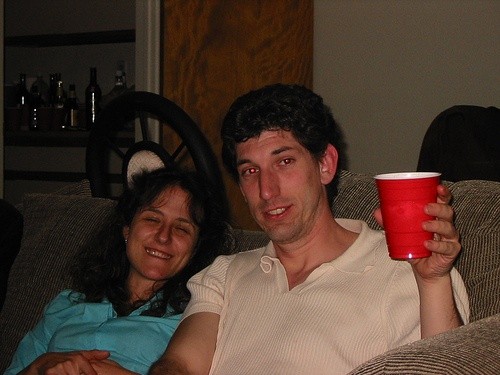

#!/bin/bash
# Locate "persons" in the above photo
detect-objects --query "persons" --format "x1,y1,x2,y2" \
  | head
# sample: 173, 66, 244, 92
3, 166, 231, 375
148, 85, 470, 375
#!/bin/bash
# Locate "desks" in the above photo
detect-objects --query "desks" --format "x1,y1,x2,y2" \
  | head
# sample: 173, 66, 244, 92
3, 129, 134, 194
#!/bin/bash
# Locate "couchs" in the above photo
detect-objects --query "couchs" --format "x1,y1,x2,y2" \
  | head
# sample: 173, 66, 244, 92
11, 171, 500, 375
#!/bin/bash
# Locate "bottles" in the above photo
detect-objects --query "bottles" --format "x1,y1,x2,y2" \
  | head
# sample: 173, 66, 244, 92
16, 73, 28, 107
85, 66, 102, 128
67, 84, 79, 129
107, 69, 131, 100
30, 72, 50, 131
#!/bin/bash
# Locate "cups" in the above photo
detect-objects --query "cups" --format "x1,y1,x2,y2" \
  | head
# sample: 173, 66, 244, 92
373, 171, 442, 259
